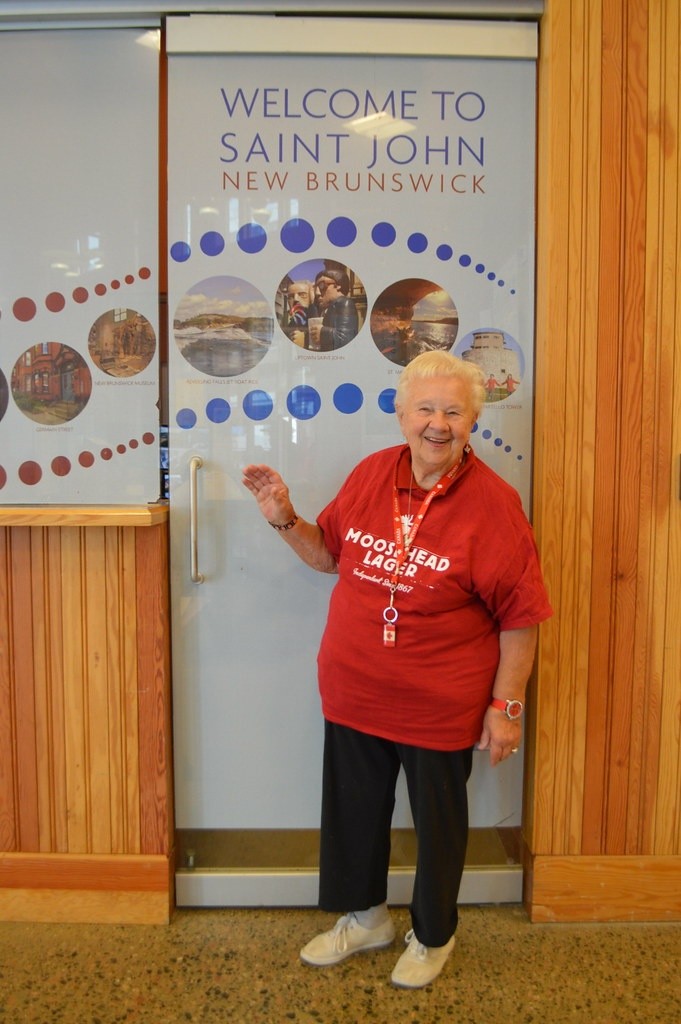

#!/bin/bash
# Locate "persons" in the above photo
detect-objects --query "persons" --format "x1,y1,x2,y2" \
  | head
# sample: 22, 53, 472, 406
484, 372, 499, 399
500, 372, 520, 396
239, 347, 555, 987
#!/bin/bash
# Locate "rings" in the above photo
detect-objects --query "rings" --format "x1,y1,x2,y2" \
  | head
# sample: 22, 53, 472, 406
512, 748, 519, 753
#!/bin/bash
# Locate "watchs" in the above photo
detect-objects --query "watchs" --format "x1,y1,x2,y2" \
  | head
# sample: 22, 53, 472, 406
491, 697, 527, 720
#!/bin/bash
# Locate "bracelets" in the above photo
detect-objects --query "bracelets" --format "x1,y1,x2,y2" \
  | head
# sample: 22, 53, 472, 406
268, 512, 298, 531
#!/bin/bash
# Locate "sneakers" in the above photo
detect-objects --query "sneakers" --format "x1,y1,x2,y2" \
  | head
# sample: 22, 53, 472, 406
391, 928, 457, 987
300, 913, 394, 965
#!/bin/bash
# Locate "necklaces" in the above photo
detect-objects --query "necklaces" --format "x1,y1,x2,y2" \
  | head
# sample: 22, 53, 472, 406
405, 456, 467, 543
